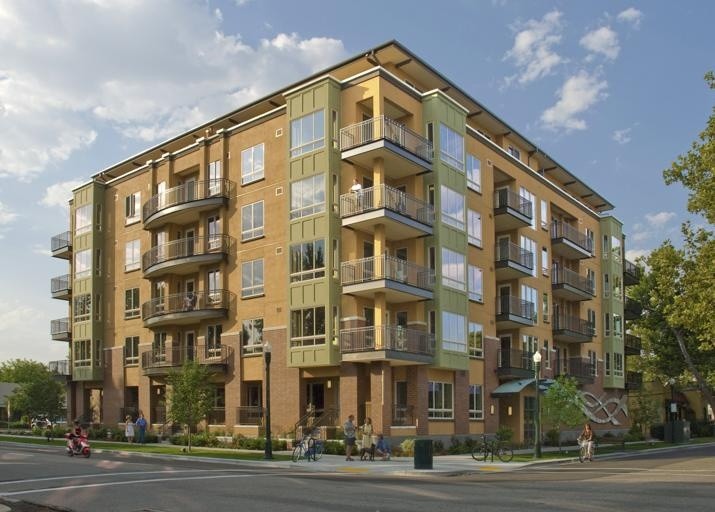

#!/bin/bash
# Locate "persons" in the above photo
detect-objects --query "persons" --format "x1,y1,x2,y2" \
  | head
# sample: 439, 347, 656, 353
124, 414, 135, 443
376, 433, 392, 461
576, 422, 594, 463
360, 417, 373, 461
44, 417, 52, 442
73, 419, 82, 451
349, 178, 362, 211
135, 411, 147, 442
342, 414, 357, 461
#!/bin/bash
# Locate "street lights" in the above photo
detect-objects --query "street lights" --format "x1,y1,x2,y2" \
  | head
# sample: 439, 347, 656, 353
667, 377, 679, 441
533, 349, 542, 458
263, 341, 274, 459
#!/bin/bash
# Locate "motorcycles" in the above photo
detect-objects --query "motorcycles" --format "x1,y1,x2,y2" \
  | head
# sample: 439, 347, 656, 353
63, 431, 93, 458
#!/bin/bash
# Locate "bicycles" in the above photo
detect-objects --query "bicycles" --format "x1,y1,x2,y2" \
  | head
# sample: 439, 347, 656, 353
471, 431, 515, 461
292, 432, 322, 462
578, 438, 596, 463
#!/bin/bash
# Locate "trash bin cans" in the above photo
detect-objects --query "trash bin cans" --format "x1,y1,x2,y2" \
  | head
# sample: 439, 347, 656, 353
414, 440, 433, 469
663, 421, 690, 443
